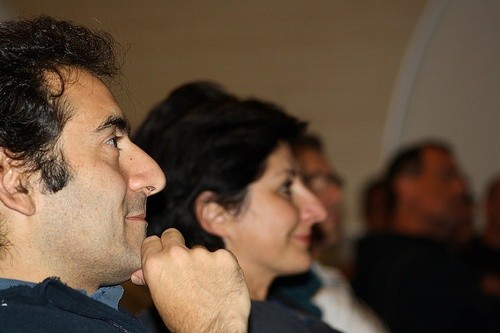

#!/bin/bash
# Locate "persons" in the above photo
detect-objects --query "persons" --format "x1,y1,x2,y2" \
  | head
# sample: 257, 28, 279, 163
263, 131, 421, 333
0, 16, 252, 333
131, 80, 344, 333
349, 138, 500, 333
